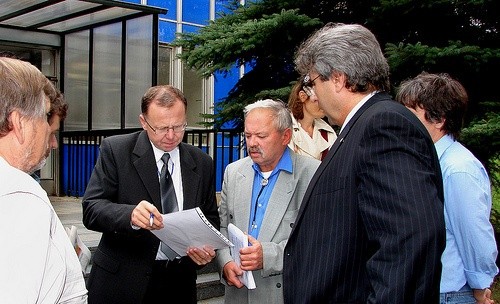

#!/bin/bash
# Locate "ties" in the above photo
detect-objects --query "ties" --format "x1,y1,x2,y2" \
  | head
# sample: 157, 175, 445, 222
159, 153, 180, 261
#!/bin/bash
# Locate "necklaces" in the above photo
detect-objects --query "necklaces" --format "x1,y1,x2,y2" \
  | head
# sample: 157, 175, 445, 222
300, 124, 313, 138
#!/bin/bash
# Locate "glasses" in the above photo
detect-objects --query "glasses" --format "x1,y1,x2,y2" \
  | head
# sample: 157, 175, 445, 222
144, 118, 187, 135
303, 74, 322, 96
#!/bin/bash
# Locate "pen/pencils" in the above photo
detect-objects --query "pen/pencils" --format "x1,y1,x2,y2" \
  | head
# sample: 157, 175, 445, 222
150, 212, 154, 227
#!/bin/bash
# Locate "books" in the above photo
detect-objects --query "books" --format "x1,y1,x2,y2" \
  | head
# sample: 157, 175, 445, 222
227, 223, 257, 289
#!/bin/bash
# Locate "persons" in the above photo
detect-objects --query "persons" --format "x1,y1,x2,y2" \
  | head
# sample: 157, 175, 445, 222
396, 71, 499, 304
81, 85, 221, 304
0, 57, 88, 304
287, 74, 338, 161
216, 99, 322, 304
283, 23, 446, 304
